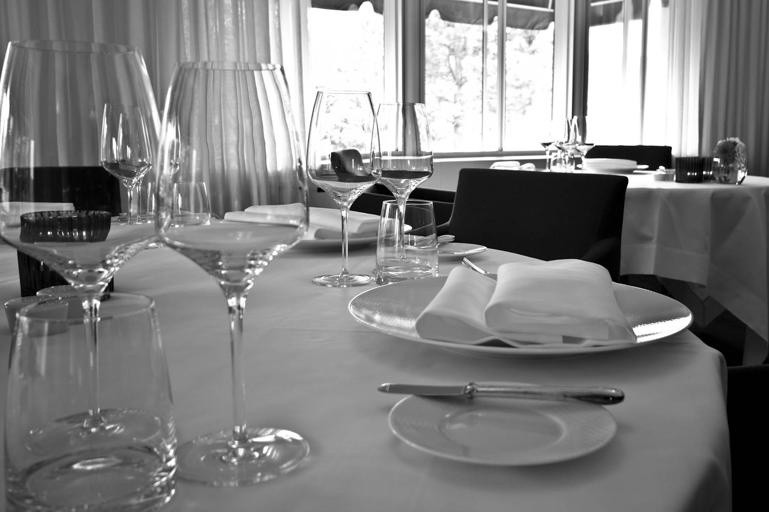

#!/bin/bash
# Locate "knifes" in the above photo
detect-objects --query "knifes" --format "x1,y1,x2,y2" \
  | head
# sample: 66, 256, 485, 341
376, 380, 625, 409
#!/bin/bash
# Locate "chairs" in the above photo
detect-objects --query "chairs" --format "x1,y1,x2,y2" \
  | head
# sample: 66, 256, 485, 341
584, 141, 673, 170
401, 166, 634, 283
0, 164, 124, 218
327, 148, 455, 228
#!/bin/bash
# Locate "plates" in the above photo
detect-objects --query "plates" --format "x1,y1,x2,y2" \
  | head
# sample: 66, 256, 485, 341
576, 163, 649, 174
436, 241, 490, 260
291, 214, 413, 250
346, 275, 695, 358
388, 394, 620, 469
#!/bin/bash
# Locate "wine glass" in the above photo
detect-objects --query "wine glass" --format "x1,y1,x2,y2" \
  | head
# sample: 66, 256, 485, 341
151, 58, 311, 485
537, 115, 596, 172
1, 34, 161, 431
304, 91, 376, 289
0, 34, 312, 510
371, 105, 444, 288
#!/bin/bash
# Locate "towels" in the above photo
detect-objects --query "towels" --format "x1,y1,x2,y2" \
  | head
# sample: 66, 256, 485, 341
224, 202, 396, 242
410, 253, 637, 349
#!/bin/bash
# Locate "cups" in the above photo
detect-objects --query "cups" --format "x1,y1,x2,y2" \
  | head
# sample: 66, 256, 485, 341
3, 293, 178, 511
376, 198, 440, 282
